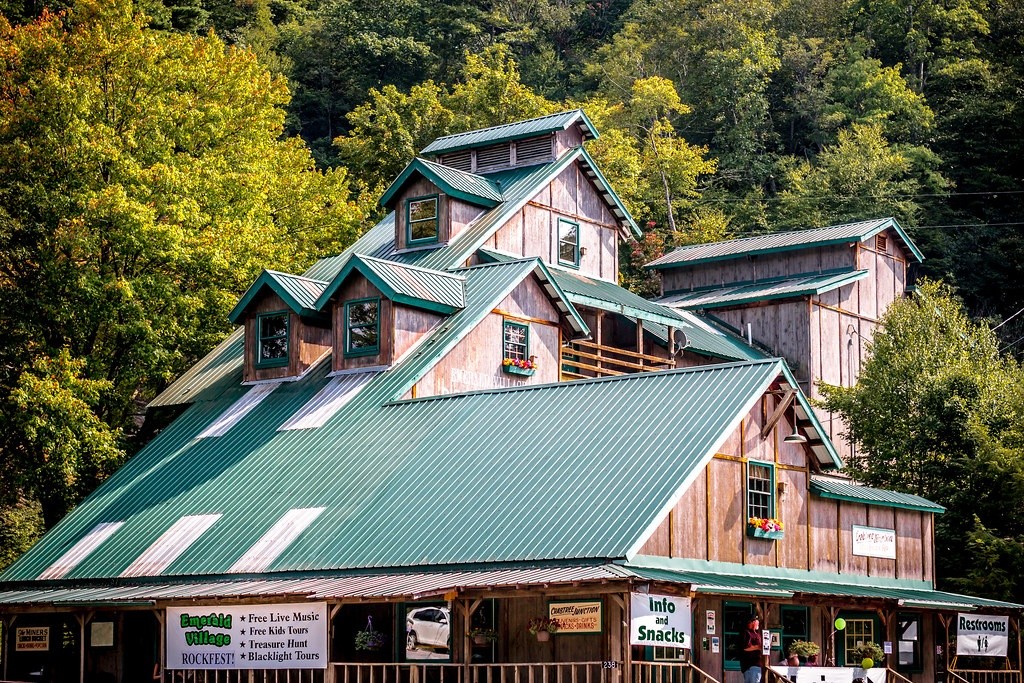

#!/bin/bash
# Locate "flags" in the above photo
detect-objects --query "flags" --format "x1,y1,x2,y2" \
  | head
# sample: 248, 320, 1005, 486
631, 592, 693, 648
957, 613, 1010, 658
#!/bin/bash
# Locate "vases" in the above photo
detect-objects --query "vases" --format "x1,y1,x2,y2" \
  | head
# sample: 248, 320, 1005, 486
473, 634, 488, 645
536, 631, 550, 641
366, 644, 380, 650
747, 526, 784, 540
504, 365, 535, 376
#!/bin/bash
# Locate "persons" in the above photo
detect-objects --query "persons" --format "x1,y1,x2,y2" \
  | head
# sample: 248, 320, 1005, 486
738, 615, 763, 683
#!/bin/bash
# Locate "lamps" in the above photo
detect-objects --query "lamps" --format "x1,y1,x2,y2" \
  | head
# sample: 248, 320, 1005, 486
783, 394, 807, 443
835, 618, 846, 630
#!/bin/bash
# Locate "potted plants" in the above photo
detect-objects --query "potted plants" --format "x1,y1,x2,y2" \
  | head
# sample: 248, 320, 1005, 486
807, 642, 820, 666
789, 639, 806, 666
853, 641, 886, 669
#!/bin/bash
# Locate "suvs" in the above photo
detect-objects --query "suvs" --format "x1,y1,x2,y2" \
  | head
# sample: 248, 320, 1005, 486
406, 607, 451, 651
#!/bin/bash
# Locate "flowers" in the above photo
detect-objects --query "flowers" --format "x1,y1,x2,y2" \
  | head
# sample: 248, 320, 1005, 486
467, 628, 497, 641
503, 359, 536, 369
527, 618, 561, 634
751, 517, 784, 533
356, 631, 384, 646
